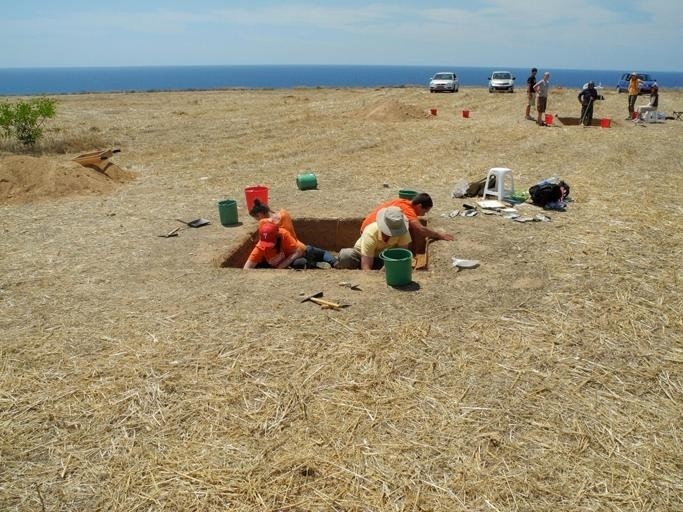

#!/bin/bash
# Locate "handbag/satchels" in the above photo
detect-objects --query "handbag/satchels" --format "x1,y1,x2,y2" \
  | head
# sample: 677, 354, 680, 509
529, 182, 562, 204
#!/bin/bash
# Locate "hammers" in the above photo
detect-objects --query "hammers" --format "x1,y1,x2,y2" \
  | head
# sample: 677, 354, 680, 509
157, 227, 180, 238
301, 290, 340, 308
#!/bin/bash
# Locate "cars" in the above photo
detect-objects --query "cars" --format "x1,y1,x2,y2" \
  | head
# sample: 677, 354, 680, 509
616, 72, 658, 95
488, 71, 517, 93
430, 71, 459, 94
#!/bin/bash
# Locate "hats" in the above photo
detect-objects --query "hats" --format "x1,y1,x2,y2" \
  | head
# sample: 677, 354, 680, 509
376, 206, 409, 237
258, 223, 278, 248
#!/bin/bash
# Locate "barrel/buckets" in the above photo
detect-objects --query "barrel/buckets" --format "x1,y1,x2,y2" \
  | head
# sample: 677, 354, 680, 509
296, 173, 318, 190
463, 111, 469, 118
545, 114, 553, 124
246, 186, 269, 212
601, 118, 611, 128
378, 248, 413, 288
631, 112, 639, 120
431, 109, 436, 116
219, 199, 238, 225
399, 189, 417, 197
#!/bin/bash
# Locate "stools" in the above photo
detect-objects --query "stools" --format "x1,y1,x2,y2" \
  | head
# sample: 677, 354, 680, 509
645, 110, 657, 123
484, 168, 515, 200
673, 111, 683, 121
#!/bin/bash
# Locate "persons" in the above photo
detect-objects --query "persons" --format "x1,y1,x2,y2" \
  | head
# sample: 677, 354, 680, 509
335, 204, 414, 271
242, 221, 309, 269
623, 72, 646, 119
360, 191, 456, 257
523, 68, 539, 120
532, 72, 551, 126
576, 80, 598, 126
249, 197, 341, 269
634, 83, 659, 124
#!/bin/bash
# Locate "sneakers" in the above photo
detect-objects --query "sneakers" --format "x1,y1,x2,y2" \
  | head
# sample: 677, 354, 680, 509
315, 260, 331, 269
333, 261, 345, 269
526, 115, 546, 126
625, 115, 641, 123
